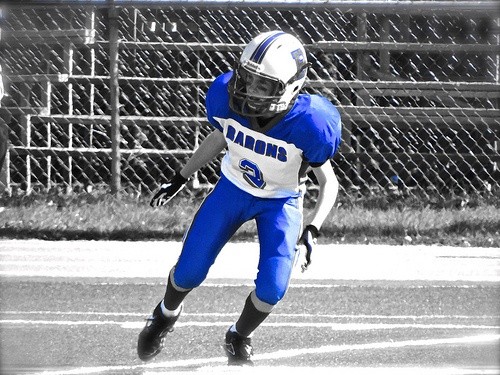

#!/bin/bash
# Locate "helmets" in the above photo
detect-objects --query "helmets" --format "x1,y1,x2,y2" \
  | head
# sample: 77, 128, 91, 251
239, 29, 308, 112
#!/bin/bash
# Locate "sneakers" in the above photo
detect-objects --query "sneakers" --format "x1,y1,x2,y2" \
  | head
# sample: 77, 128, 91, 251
137, 303, 182, 361
222, 326, 255, 367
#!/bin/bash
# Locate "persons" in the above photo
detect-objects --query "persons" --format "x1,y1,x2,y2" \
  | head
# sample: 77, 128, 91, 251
137, 30, 341, 367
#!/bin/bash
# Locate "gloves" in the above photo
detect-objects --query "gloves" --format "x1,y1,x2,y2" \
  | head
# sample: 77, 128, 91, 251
295, 225, 320, 274
151, 170, 188, 207
